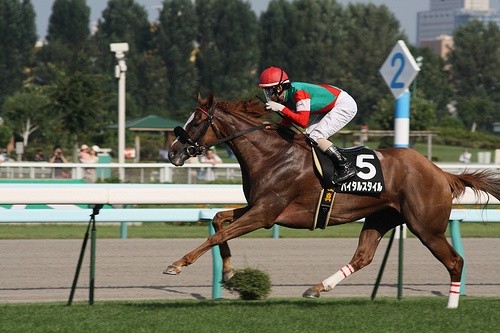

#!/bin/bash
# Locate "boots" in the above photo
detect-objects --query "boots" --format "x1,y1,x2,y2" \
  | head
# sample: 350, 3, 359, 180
323, 143, 358, 185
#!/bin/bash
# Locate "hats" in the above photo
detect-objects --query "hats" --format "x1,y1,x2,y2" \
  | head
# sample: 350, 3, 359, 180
80, 144, 89, 150
91, 145, 100, 153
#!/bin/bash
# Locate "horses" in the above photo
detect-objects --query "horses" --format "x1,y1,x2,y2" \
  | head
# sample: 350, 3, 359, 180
162, 92, 500, 309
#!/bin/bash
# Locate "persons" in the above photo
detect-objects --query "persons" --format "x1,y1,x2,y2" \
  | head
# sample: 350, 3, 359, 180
198, 149, 222, 181
32, 143, 100, 184
256, 67, 358, 186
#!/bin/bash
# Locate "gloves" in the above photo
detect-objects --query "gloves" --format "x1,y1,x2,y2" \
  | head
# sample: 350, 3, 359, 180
266, 101, 285, 111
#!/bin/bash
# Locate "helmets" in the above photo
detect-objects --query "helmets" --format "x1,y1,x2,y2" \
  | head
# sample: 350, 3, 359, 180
259, 66, 290, 88
